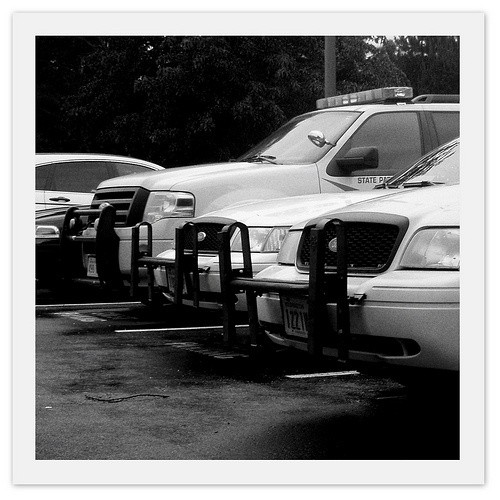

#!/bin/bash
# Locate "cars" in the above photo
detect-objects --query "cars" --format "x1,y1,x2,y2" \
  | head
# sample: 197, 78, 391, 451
150, 138, 458, 310
254, 182, 461, 371
34, 152, 166, 246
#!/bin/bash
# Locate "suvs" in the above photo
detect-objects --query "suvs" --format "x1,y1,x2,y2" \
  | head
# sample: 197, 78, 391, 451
82, 87, 459, 282
